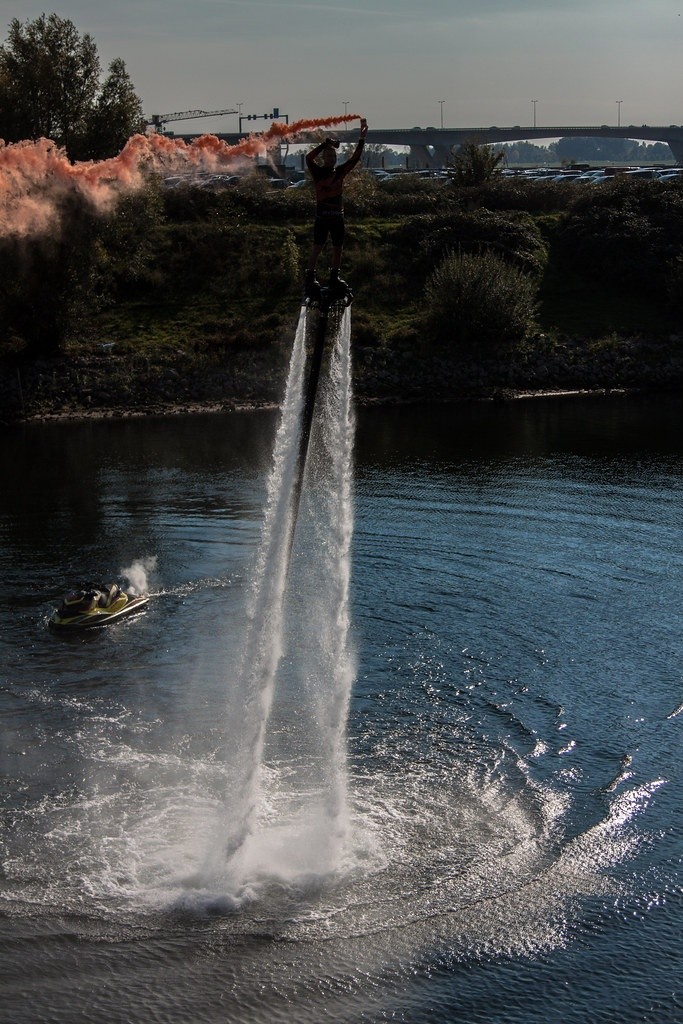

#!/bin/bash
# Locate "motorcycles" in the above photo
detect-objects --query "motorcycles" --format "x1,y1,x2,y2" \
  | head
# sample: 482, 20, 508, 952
47, 580, 151, 633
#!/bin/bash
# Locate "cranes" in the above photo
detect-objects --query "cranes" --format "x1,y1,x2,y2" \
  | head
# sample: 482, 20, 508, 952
146, 108, 239, 135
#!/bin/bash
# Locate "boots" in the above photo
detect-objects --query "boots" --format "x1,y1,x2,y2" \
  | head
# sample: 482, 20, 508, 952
328, 266, 348, 291
303, 269, 321, 290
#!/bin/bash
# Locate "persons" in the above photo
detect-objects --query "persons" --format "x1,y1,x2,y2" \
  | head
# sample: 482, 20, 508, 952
304, 124, 370, 307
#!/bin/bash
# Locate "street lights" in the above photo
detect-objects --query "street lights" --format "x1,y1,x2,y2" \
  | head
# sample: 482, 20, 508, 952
532, 100, 539, 127
342, 101, 352, 131
616, 100, 623, 127
438, 100, 447, 128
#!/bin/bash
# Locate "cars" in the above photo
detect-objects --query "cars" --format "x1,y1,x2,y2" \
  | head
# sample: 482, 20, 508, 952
162, 163, 683, 190
